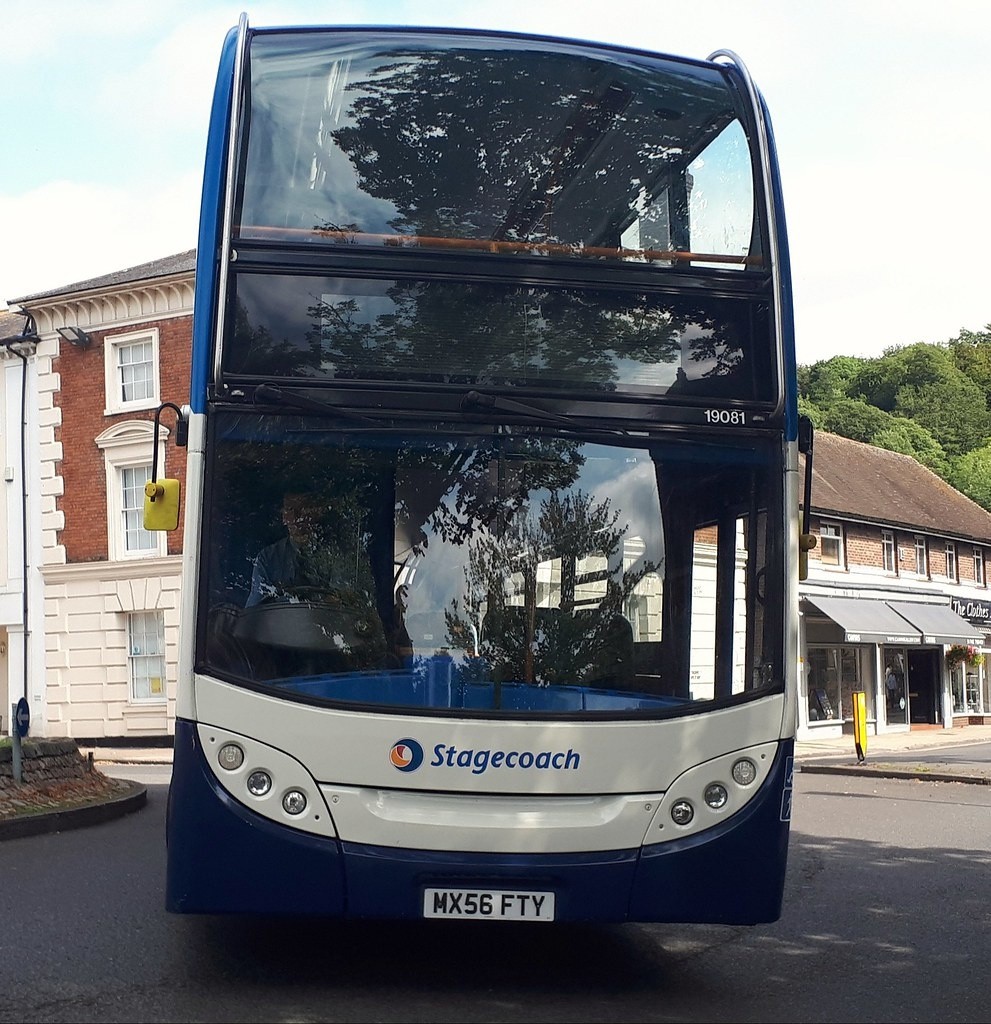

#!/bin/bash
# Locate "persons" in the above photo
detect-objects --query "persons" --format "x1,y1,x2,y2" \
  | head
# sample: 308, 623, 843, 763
245, 486, 376, 608
884, 666, 899, 709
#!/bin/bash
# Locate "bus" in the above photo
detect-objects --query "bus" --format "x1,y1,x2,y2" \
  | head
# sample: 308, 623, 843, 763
141, 11, 817, 927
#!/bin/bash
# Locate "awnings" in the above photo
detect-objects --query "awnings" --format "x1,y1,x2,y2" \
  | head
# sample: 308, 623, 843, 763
885, 600, 986, 646
804, 597, 922, 646
